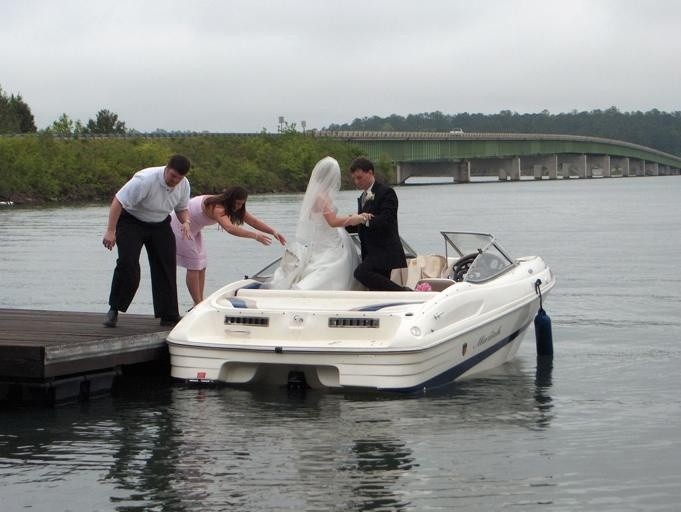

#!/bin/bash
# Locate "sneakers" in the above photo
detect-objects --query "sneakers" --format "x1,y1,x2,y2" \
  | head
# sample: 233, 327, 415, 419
159, 317, 182, 327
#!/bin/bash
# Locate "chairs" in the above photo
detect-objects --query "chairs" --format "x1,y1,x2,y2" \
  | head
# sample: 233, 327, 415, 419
414, 278, 456, 293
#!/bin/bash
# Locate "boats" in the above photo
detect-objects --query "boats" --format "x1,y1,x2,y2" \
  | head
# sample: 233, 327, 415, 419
166, 232, 556, 393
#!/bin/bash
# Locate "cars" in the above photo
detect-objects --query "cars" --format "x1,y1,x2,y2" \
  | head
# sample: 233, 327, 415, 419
450, 128, 463, 135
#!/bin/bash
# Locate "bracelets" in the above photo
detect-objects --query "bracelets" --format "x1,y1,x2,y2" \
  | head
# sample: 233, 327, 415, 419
186, 219, 194, 225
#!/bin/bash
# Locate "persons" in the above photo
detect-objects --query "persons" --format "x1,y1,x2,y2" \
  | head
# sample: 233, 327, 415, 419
101, 154, 193, 328
344, 157, 415, 291
258, 156, 375, 291
170, 185, 289, 306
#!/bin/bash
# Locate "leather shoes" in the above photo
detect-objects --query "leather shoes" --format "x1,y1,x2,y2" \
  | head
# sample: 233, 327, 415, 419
103, 306, 119, 328
